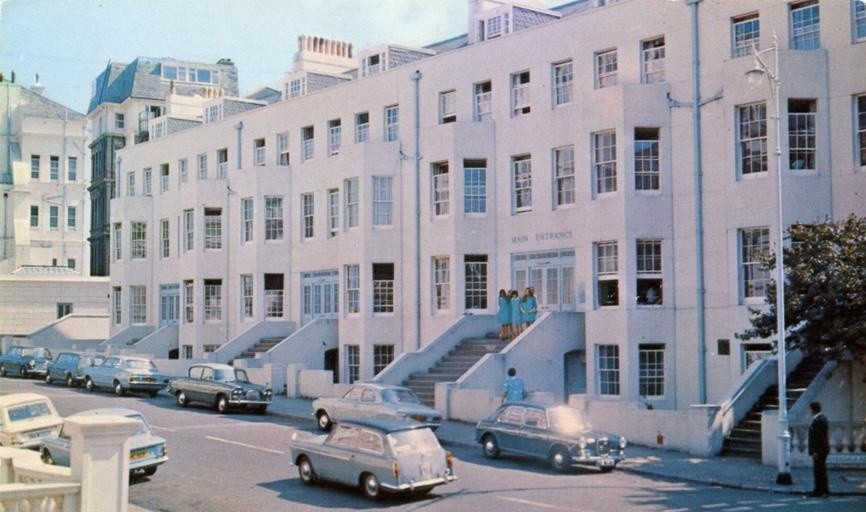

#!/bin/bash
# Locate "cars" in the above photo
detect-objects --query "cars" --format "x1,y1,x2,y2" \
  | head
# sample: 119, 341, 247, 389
0, 342, 172, 487
164, 360, 275, 411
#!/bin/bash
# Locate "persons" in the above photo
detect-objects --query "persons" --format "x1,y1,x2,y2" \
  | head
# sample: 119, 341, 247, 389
496, 286, 539, 340
499, 367, 527, 405
808, 401, 830, 497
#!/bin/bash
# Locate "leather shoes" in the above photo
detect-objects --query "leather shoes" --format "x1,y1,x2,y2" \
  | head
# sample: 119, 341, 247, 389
807, 491, 832, 498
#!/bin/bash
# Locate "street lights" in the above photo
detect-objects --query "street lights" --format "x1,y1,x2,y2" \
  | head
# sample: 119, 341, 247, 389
743, 28, 798, 489
